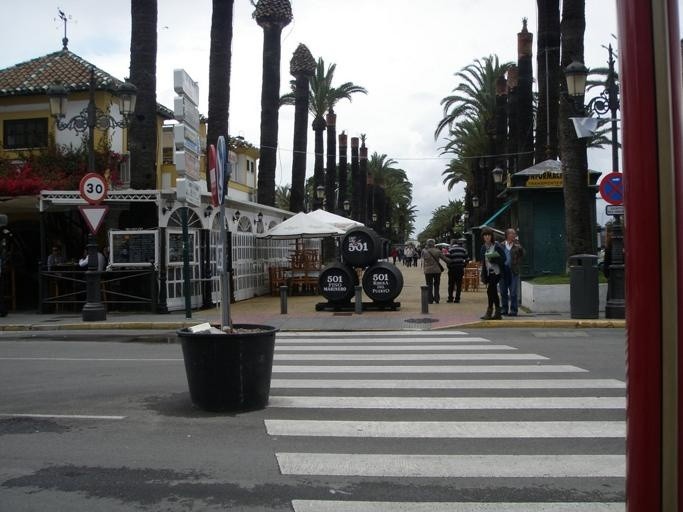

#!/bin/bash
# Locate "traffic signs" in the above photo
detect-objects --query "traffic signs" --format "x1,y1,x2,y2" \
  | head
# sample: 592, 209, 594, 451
80, 173, 108, 205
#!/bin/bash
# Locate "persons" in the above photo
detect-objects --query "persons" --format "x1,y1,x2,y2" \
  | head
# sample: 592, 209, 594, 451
390, 238, 468, 304
499, 228, 523, 316
47, 239, 109, 270
477, 228, 506, 320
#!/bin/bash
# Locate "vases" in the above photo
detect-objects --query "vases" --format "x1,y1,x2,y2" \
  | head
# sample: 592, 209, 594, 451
177, 322, 280, 413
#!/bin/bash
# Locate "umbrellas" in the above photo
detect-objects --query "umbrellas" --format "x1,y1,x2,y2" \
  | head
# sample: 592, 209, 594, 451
254, 212, 345, 277
306, 209, 363, 229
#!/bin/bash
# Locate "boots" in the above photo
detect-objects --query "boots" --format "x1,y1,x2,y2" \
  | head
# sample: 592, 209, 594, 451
480, 306, 503, 320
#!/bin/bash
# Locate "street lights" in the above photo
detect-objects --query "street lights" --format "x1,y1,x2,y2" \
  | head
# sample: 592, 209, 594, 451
562, 42, 622, 319
491, 167, 508, 191
304, 180, 391, 230
457, 196, 481, 227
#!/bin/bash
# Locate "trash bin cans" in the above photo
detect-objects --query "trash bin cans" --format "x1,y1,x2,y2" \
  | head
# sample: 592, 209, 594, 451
570, 254, 600, 318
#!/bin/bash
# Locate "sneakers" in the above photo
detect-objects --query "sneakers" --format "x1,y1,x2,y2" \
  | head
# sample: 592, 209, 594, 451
428, 296, 460, 304
501, 307, 518, 316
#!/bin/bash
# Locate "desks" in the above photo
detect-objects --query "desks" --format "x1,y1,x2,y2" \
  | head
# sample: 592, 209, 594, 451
53, 262, 80, 313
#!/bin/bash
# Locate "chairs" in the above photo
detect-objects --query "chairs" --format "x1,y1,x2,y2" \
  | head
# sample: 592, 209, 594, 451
458, 260, 487, 292
269, 261, 326, 296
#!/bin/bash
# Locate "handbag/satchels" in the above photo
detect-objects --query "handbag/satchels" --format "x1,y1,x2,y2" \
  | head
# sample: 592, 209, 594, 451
438, 262, 444, 272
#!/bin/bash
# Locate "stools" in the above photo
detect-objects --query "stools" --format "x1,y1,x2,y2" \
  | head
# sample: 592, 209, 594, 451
46, 276, 108, 315
